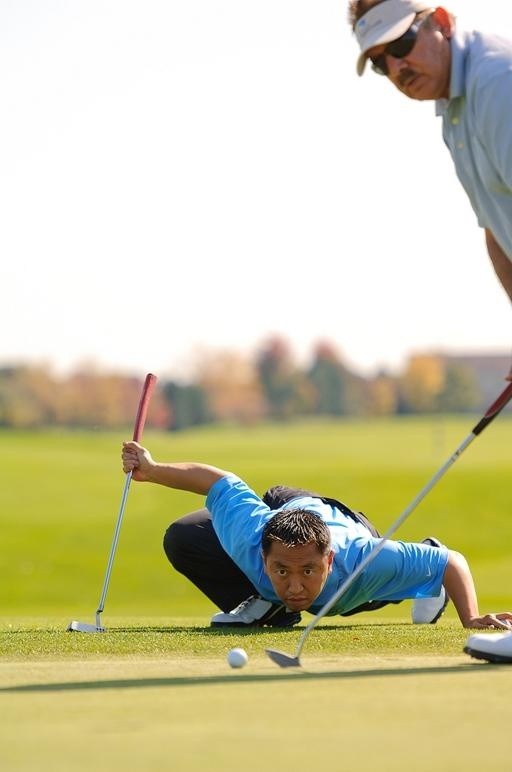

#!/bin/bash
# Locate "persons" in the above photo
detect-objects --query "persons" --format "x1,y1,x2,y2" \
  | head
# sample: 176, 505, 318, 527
121, 440, 511, 629
346, 1, 511, 663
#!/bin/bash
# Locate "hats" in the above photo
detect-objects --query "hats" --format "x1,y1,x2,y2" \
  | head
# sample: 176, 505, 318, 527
354, 0, 429, 76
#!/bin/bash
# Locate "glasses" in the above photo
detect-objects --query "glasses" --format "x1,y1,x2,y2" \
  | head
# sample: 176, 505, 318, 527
370, 16, 428, 76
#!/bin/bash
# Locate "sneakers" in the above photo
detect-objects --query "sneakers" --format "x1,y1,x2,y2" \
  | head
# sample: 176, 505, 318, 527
210, 595, 302, 628
463, 628, 512, 663
411, 537, 450, 625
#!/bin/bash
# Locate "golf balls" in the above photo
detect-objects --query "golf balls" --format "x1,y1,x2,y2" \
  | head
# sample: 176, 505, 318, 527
229, 648, 246, 669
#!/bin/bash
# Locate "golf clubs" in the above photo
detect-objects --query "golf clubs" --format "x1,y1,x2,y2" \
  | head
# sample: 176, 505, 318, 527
68, 374, 157, 633
265, 383, 512, 668
